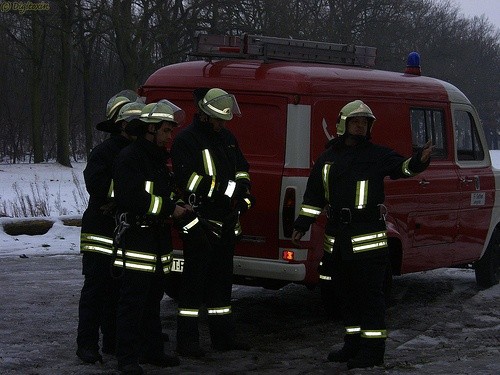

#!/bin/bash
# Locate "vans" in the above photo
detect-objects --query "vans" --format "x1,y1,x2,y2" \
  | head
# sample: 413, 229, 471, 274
140, 33, 500, 308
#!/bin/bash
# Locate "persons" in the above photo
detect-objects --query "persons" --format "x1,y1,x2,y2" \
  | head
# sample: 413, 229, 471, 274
292, 99, 437, 369
76, 88, 250, 375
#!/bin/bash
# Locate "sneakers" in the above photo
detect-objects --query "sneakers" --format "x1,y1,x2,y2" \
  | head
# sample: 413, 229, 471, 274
102, 346, 115, 354
175, 345, 205, 357
212, 337, 249, 350
76, 347, 101, 362
139, 349, 179, 366
347, 350, 385, 368
118, 359, 143, 375
327, 343, 358, 362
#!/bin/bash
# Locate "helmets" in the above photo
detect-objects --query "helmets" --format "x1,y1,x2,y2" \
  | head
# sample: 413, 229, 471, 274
115, 102, 144, 123
139, 100, 185, 124
336, 102, 376, 136
106, 90, 139, 120
198, 88, 243, 121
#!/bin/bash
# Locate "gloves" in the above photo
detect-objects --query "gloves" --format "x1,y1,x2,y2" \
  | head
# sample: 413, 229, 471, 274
174, 212, 221, 258
